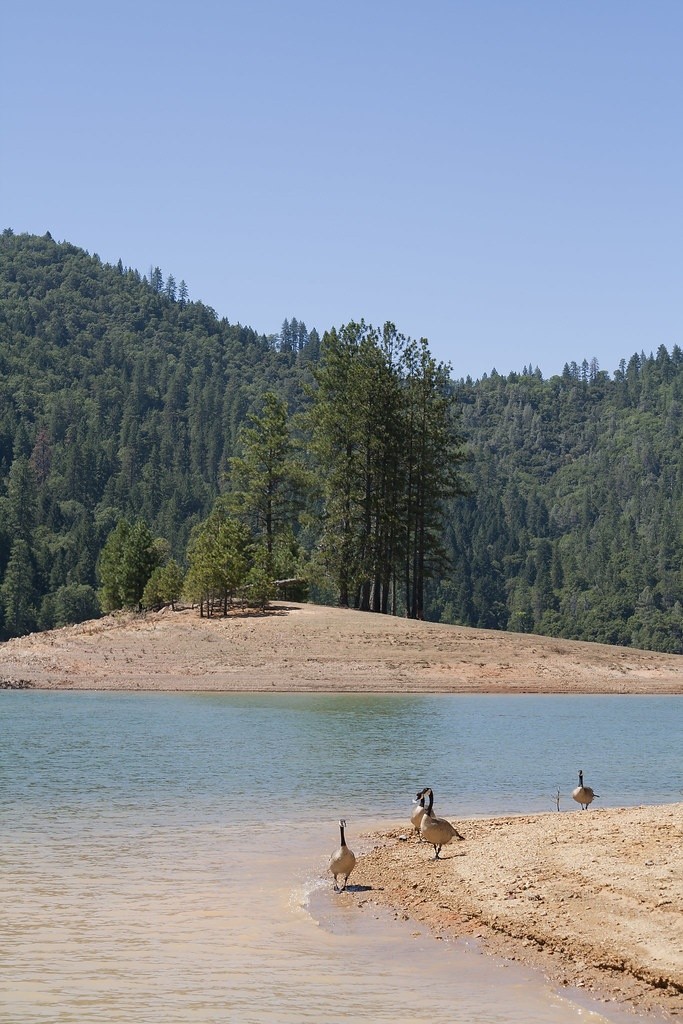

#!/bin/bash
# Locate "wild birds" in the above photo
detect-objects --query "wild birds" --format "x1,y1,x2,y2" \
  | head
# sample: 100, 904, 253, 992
328, 819, 356, 894
572, 768, 600, 810
409, 790, 436, 836
419, 787, 466, 860
